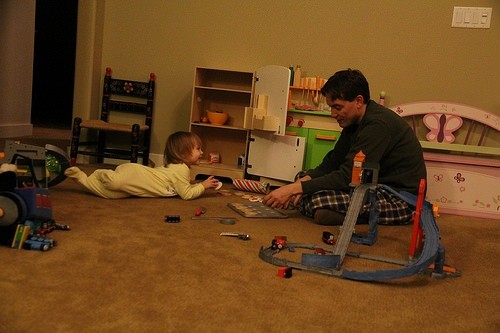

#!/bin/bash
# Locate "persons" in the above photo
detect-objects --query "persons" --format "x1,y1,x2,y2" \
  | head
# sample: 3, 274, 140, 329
263, 68, 427, 224
64, 131, 219, 200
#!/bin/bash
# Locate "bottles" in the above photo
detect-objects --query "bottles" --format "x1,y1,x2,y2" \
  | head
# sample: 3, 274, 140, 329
287, 64, 302, 87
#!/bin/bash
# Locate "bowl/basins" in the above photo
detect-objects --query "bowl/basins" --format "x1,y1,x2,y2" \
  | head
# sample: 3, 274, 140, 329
207, 112, 229, 126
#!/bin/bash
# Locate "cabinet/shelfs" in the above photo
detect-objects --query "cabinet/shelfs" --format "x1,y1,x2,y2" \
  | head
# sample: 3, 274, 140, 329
187, 66, 341, 183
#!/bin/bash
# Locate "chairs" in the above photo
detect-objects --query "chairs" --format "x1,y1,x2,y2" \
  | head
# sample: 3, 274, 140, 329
70, 67, 155, 165
